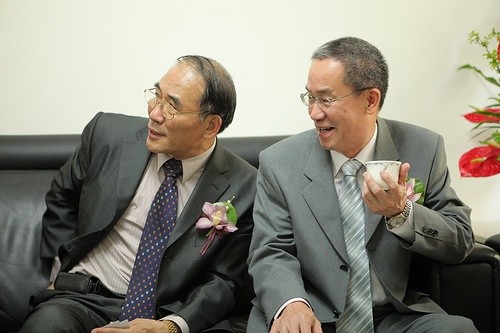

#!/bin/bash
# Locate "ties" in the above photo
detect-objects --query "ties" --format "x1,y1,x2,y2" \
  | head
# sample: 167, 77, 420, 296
337, 160, 374, 333
118, 157, 183, 321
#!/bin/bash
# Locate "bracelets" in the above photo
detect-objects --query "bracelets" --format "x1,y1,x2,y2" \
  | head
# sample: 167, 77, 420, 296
163, 320, 177, 333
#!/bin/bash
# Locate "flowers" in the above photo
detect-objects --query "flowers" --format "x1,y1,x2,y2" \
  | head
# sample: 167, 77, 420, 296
405, 175, 425, 206
455, 26, 500, 179
194, 194, 239, 256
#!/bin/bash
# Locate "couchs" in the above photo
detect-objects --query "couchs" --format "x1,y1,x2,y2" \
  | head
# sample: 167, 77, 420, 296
0, 134, 500, 333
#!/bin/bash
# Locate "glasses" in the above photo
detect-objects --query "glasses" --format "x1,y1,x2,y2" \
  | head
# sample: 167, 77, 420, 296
300, 87, 372, 111
144, 87, 209, 120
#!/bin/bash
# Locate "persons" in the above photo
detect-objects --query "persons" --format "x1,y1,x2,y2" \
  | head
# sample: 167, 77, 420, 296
245, 37, 480, 333
16, 56, 259, 333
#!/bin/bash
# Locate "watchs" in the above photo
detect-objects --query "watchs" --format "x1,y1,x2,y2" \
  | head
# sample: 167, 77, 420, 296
386, 198, 411, 228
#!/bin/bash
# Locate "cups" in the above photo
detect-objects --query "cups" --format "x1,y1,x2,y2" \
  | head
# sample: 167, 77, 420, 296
365, 161, 402, 190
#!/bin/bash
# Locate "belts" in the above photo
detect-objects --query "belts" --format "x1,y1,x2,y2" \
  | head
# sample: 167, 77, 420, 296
91, 280, 124, 299
